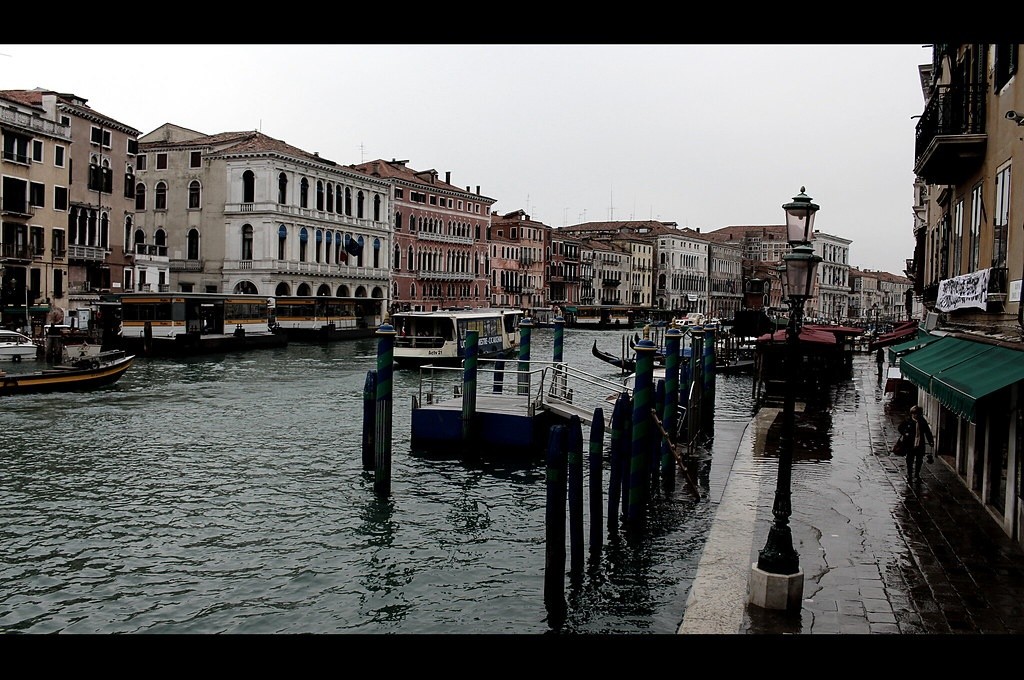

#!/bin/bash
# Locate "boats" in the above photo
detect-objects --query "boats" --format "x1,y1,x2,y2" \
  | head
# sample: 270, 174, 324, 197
0, 328, 102, 364
114, 292, 289, 357
0, 322, 135, 395
592, 332, 756, 373
559, 305, 636, 330
272, 296, 390, 338
391, 308, 524, 369
670, 312, 722, 329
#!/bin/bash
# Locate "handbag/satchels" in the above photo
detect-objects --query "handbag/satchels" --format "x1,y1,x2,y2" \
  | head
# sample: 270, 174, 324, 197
892, 435, 906, 456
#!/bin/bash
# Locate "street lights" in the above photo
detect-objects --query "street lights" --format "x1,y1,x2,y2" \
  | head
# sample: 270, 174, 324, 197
758, 185, 823, 574
871, 301, 879, 336
836, 304, 842, 326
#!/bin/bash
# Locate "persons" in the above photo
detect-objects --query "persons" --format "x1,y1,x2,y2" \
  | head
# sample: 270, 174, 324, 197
898, 406, 934, 483
875, 348, 885, 377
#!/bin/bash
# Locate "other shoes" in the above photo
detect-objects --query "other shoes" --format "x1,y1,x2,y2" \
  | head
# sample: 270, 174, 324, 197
907, 475, 913, 482
914, 474, 922, 481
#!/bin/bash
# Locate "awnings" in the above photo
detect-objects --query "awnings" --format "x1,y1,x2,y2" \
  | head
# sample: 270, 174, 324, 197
871, 319, 920, 352
888, 336, 941, 366
900, 336, 1024, 427
